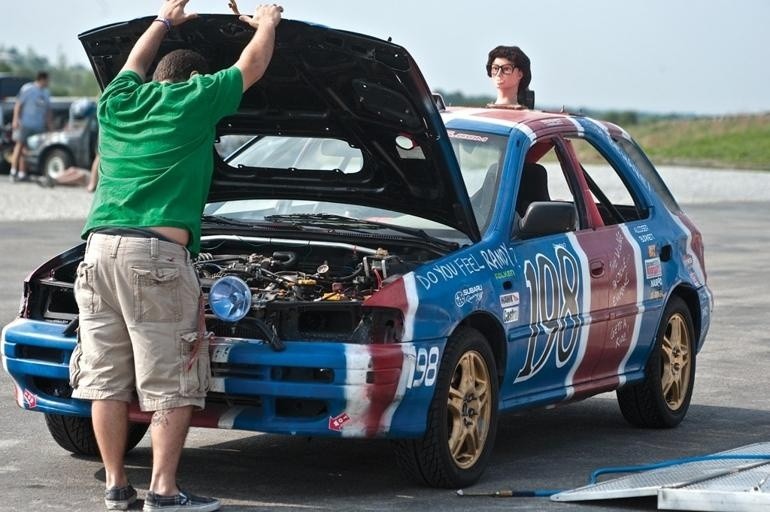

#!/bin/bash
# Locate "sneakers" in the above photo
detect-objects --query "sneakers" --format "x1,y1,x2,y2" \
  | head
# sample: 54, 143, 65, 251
142, 485, 220, 512
104, 481, 137, 510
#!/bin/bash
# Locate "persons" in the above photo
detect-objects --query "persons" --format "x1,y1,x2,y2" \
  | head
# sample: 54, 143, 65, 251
86, 153, 100, 193
9, 70, 54, 182
485, 46, 532, 111
69, 0, 285, 512
30, 164, 101, 190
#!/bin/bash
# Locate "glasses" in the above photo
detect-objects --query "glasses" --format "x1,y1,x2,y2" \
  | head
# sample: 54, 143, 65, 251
487, 63, 518, 75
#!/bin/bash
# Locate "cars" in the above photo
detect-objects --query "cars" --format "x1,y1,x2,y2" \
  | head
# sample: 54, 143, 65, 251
0, 71, 103, 184
0, 11, 720, 490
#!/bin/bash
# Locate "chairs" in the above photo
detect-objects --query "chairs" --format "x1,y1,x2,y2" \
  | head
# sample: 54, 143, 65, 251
470, 162, 550, 240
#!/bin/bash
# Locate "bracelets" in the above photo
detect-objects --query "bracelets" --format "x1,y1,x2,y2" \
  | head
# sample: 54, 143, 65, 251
154, 16, 172, 33
